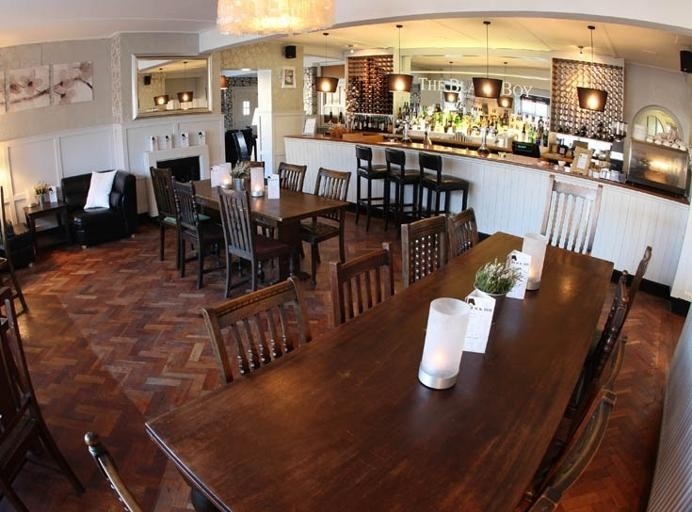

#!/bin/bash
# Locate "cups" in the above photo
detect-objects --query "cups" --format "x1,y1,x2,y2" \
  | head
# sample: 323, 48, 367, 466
220, 161, 232, 189
250, 166, 264, 197
520, 228, 550, 288
418, 296, 474, 392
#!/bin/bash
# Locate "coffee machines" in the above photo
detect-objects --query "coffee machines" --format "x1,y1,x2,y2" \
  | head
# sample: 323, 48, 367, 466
609, 119, 628, 172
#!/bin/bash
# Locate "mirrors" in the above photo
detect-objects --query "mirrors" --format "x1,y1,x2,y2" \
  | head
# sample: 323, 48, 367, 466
132, 53, 213, 120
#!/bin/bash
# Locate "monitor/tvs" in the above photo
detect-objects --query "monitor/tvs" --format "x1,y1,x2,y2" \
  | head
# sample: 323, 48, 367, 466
512, 141, 540, 158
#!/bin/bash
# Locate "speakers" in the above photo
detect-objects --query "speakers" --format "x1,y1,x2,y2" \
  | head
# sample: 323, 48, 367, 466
144, 75, 151, 85
285, 46, 296, 58
681, 51, 692, 73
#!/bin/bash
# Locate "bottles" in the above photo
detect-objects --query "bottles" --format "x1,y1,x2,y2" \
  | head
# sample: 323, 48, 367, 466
393, 102, 551, 130
328, 111, 345, 127
351, 114, 391, 131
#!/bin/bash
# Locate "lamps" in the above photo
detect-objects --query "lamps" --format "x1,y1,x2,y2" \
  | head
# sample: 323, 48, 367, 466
315, 33, 338, 92
176, 62, 193, 102
153, 68, 168, 106
220, 75, 228, 89
216, 0, 336, 36
386, 21, 607, 112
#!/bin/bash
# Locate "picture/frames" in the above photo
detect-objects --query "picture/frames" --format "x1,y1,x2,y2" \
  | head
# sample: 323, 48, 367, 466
281, 65, 297, 88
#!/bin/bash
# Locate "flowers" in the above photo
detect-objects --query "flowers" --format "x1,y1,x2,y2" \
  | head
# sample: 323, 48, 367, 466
0, 62, 92, 106
